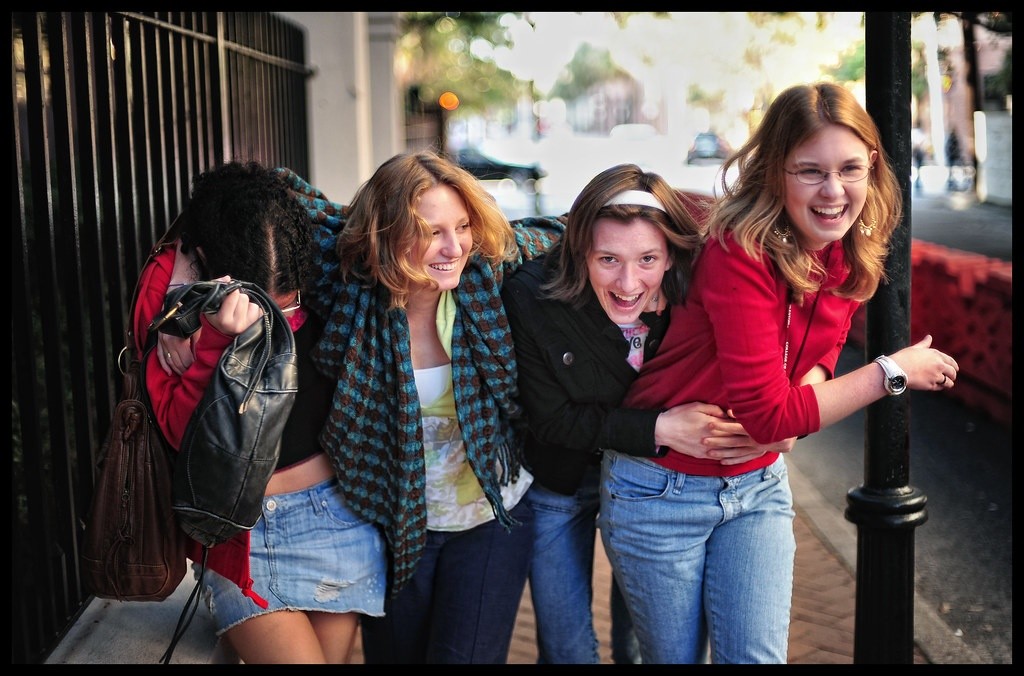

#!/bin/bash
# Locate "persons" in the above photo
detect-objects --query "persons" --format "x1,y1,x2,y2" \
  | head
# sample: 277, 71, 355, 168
599, 81, 960, 666
132, 157, 389, 663
499, 161, 767, 668
270, 147, 665, 667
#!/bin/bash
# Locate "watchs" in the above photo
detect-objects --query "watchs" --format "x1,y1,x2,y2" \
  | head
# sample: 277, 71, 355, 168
873, 354, 909, 397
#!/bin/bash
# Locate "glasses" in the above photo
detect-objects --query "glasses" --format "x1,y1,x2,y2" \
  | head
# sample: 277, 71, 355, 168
780, 163, 876, 184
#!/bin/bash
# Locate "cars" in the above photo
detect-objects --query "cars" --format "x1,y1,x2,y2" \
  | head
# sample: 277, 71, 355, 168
685, 131, 735, 168
450, 139, 551, 198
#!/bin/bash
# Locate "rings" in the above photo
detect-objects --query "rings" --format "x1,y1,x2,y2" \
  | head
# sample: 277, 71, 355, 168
167, 353, 171, 357
937, 374, 947, 385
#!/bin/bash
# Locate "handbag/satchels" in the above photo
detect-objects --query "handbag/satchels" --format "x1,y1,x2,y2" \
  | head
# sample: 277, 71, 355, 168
92, 399, 184, 603
147, 277, 298, 550
